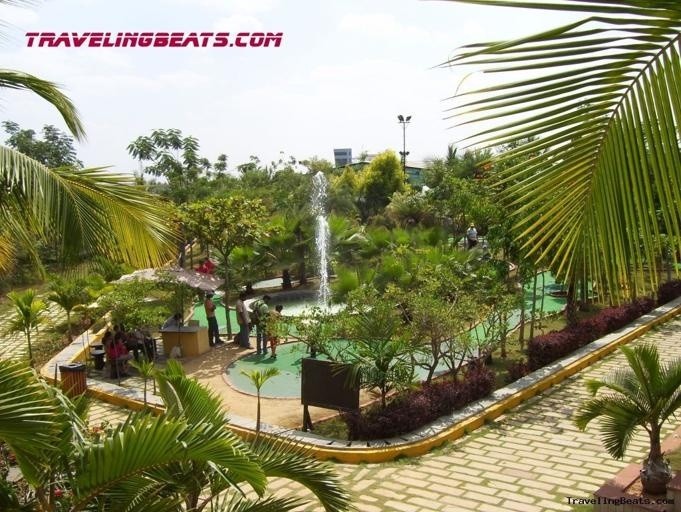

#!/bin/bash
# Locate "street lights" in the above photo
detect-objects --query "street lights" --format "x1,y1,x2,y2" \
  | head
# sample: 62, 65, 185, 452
398, 114, 411, 178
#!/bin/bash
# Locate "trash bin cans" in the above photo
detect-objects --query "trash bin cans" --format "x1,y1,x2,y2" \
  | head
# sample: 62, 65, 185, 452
59, 363, 87, 397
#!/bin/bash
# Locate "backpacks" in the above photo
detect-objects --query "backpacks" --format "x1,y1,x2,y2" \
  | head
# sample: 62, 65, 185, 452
250, 301, 265, 325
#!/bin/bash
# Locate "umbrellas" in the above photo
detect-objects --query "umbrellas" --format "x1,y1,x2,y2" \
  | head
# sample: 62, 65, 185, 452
104, 258, 226, 323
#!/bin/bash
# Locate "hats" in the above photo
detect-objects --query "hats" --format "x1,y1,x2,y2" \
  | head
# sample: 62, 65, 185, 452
207, 291, 217, 295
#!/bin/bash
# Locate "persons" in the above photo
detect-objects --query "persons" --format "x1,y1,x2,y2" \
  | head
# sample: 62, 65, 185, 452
235, 293, 252, 347
254, 294, 272, 355
205, 289, 225, 346
267, 303, 283, 359
163, 312, 182, 329
99, 322, 157, 376
467, 223, 479, 251
